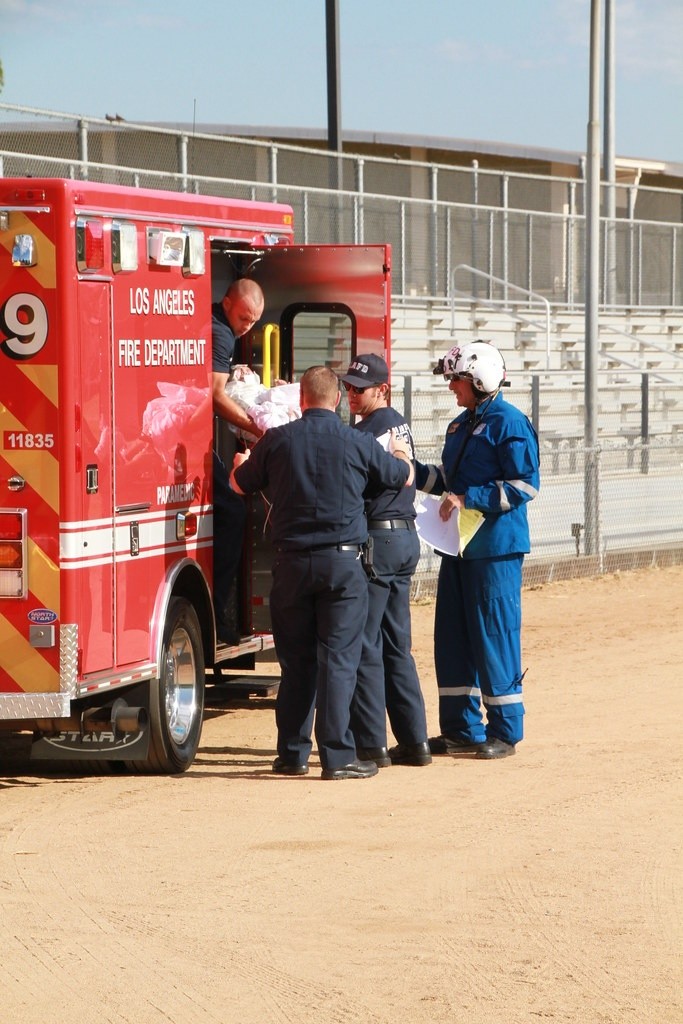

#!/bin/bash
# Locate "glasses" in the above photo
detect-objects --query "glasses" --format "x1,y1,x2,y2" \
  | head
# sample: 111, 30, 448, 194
342, 381, 376, 394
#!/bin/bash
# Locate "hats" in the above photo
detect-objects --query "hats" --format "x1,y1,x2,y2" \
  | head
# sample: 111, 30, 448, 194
342, 353, 388, 388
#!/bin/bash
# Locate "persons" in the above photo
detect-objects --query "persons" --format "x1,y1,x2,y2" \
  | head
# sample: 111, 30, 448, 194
410, 342, 541, 757
207, 280, 298, 646
230, 367, 412, 780
340, 353, 433, 768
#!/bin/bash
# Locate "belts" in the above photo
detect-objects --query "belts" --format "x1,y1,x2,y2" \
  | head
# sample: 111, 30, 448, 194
368, 520, 415, 529
277, 545, 360, 552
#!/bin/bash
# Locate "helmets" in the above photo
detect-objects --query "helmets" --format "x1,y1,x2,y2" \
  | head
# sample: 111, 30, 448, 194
433, 339, 506, 398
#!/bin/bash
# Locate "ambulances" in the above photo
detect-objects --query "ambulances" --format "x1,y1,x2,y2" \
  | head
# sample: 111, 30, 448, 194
1, 178, 393, 777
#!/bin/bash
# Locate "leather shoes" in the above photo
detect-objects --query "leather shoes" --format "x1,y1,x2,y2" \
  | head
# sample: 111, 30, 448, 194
476, 736, 515, 758
428, 735, 486, 754
356, 747, 391, 767
321, 756, 378, 779
388, 744, 432, 766
273, 757, 309, 775
